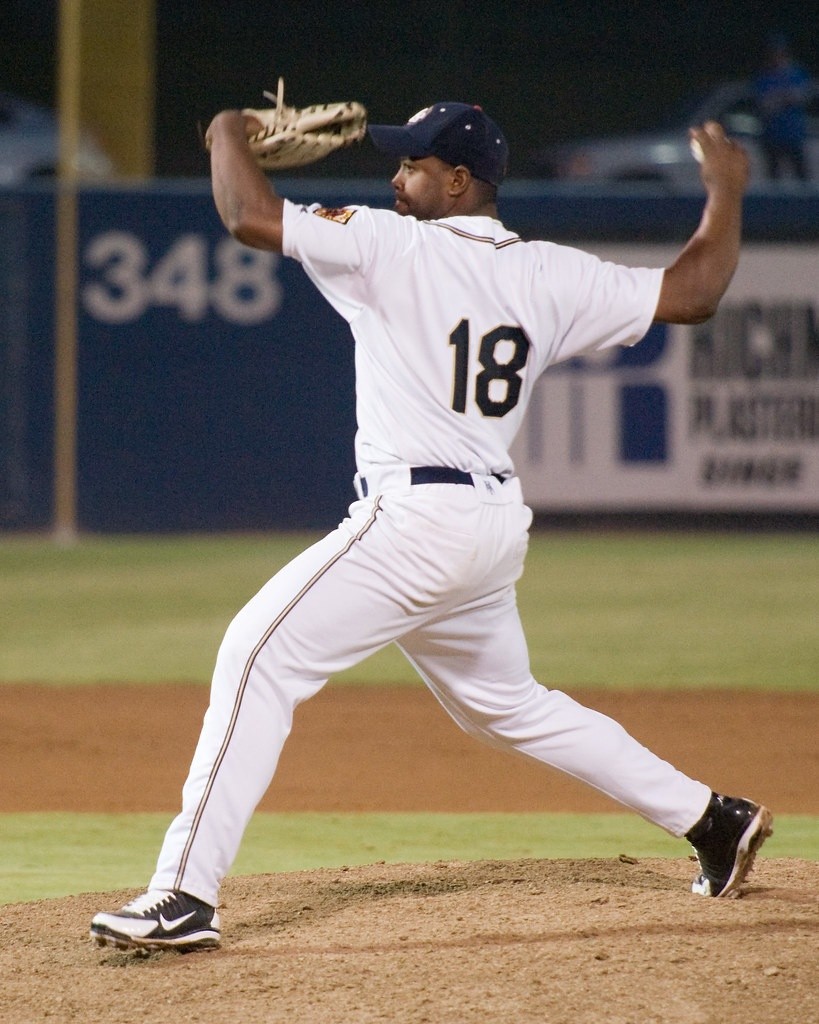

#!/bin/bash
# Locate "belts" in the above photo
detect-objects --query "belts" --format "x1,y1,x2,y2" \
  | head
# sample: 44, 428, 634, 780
359, 466, 505, 496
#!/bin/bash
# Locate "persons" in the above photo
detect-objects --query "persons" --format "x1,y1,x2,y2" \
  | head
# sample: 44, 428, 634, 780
542, 35, 817, 180
92, 105, 776, 957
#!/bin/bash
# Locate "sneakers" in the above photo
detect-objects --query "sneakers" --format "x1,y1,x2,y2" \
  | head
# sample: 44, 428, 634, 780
692, 796, 773, 899
89, 889, 220, 951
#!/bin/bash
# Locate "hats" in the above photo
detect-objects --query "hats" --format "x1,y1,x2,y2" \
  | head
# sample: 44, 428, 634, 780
367, 101, 509, 186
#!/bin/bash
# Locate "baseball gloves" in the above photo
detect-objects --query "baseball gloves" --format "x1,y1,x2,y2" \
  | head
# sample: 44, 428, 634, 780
204, 75, 367, 169
